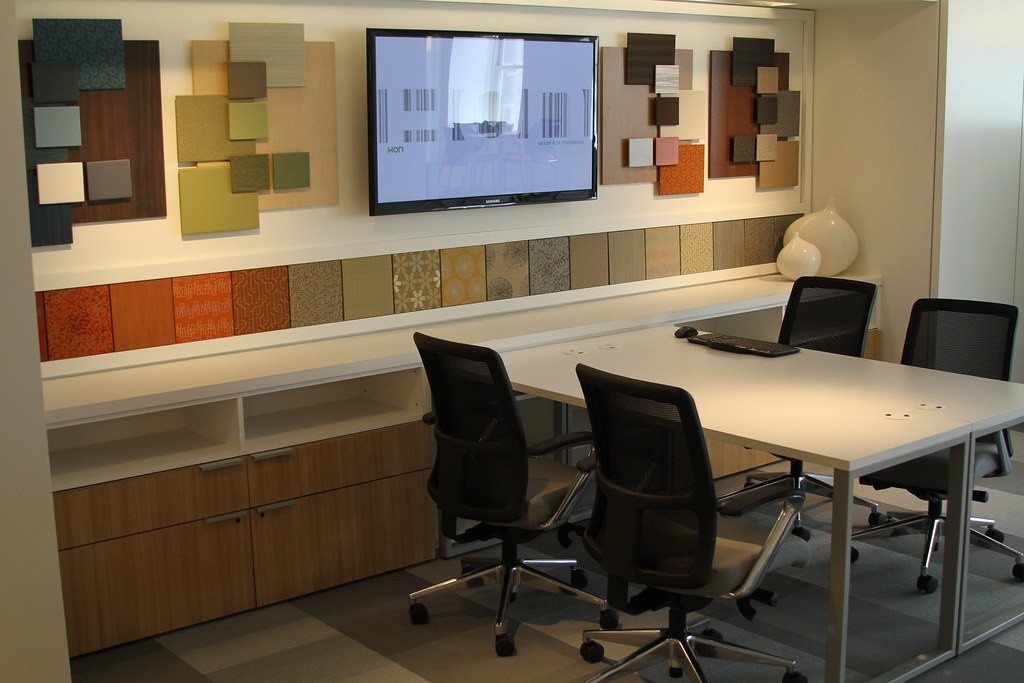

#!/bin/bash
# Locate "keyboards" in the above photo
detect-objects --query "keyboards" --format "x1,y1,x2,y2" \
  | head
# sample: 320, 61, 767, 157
687, 334, 801, 358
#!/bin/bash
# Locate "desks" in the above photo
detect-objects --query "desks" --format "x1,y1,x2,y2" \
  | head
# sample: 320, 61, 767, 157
497, 324, 1024, 683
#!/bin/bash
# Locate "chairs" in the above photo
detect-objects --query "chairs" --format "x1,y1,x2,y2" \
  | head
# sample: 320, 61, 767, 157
714, 275, 888, 543
850, 297, 1024, 595
408, 332, 621, 658
574, 361, 812, 683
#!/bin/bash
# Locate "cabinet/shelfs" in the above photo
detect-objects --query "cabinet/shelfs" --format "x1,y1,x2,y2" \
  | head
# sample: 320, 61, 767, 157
52, 418, 437, 659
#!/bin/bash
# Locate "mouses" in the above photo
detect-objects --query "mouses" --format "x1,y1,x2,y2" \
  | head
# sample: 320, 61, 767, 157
674, 326, 698, 338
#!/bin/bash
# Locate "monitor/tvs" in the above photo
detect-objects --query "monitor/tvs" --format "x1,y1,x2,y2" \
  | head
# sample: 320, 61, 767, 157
365, 28, 599, 216
